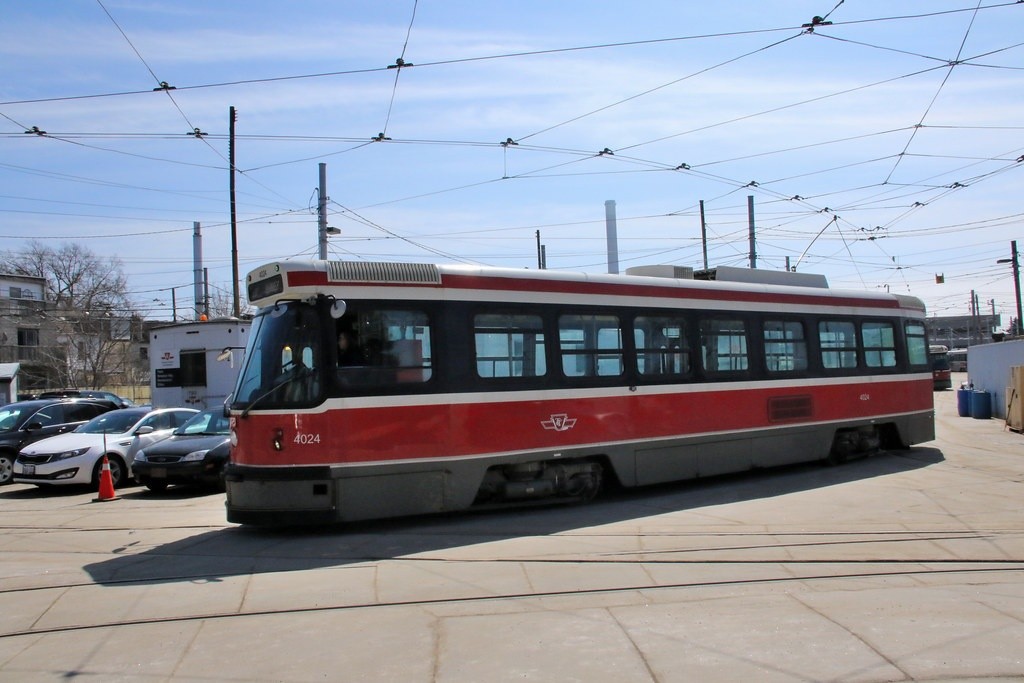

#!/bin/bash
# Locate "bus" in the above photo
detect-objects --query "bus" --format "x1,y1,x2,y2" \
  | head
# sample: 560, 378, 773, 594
948, 347, 967, 372
929, 344, 952, 391
221, 214, 934, 526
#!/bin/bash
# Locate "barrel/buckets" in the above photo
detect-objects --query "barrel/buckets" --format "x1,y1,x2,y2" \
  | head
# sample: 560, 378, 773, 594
957, 389, 992, 419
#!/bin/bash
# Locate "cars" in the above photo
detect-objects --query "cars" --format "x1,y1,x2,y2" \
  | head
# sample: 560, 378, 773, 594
0, 388, 153, 487
131, 403, 234, 494
12, 403, 229, 497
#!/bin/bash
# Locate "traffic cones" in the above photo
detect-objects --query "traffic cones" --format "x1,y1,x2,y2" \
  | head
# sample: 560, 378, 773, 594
92, 454, 122, 502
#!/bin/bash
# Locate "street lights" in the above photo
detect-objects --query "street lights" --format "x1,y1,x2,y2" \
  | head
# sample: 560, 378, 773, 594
997, 240, 1024, 335
